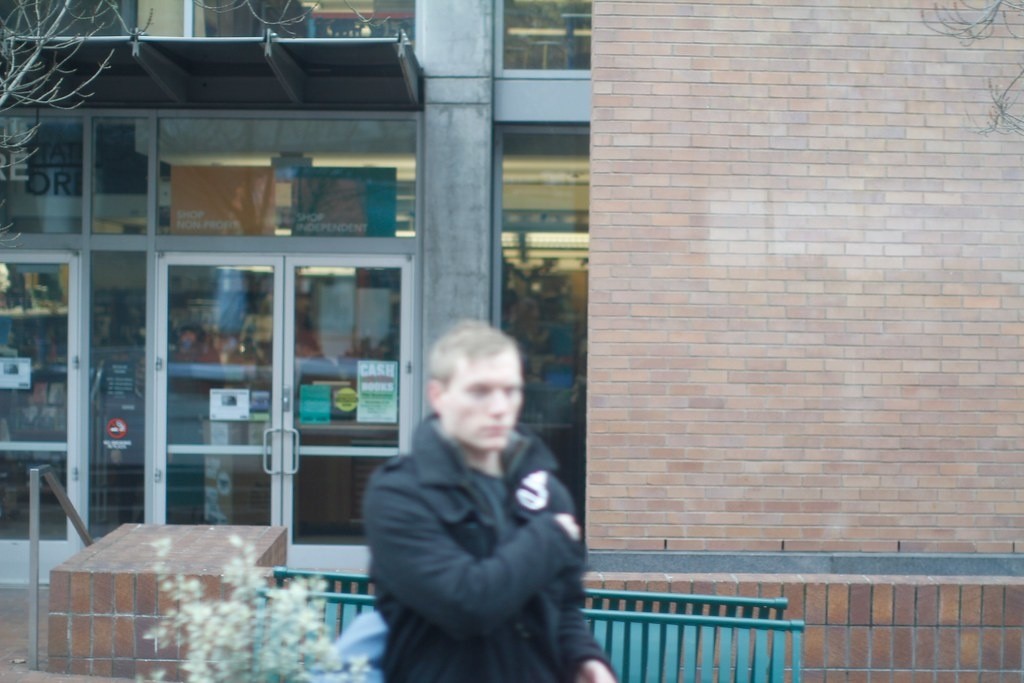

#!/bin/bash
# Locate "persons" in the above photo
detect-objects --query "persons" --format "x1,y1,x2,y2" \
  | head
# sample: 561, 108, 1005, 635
362, 315, 625, 683
